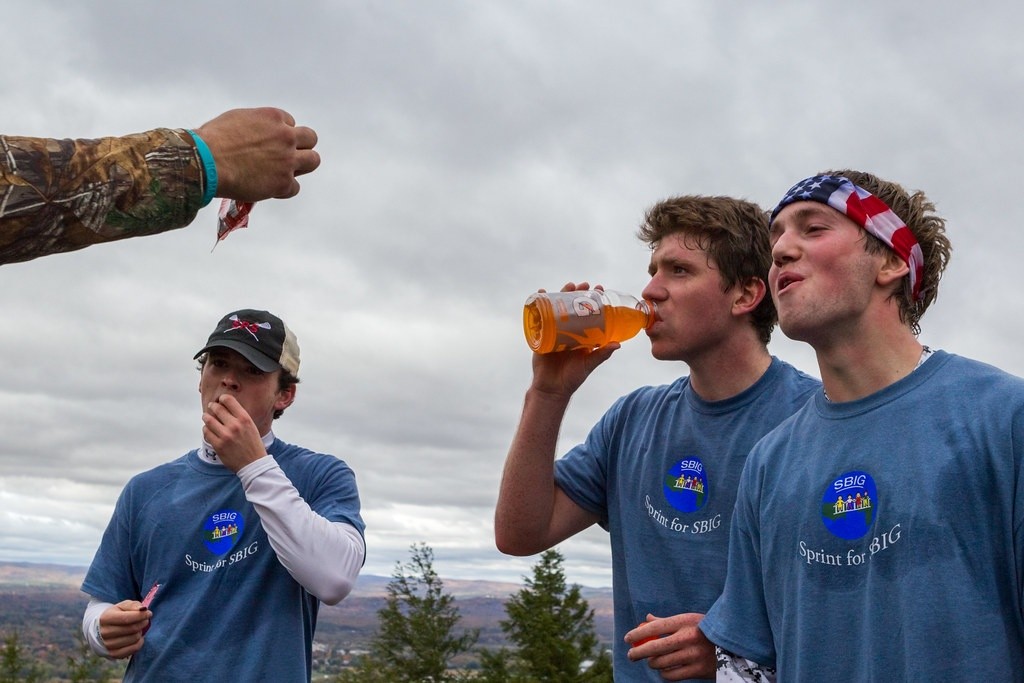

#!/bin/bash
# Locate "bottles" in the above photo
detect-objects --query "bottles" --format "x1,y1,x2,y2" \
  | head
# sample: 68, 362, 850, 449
523, 292, 659, 355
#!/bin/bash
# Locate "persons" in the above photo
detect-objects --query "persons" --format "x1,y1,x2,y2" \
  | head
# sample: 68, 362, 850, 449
494, 195, 822, 683
81, 310, 366, 683
0, 107, 320, 265
698, 170, 1024, 682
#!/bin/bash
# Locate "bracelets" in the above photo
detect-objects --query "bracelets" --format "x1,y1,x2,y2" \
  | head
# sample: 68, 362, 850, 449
187, 130, 218, 209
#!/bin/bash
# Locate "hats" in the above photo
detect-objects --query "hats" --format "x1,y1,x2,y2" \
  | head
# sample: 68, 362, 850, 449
193, 309, 299, 378
